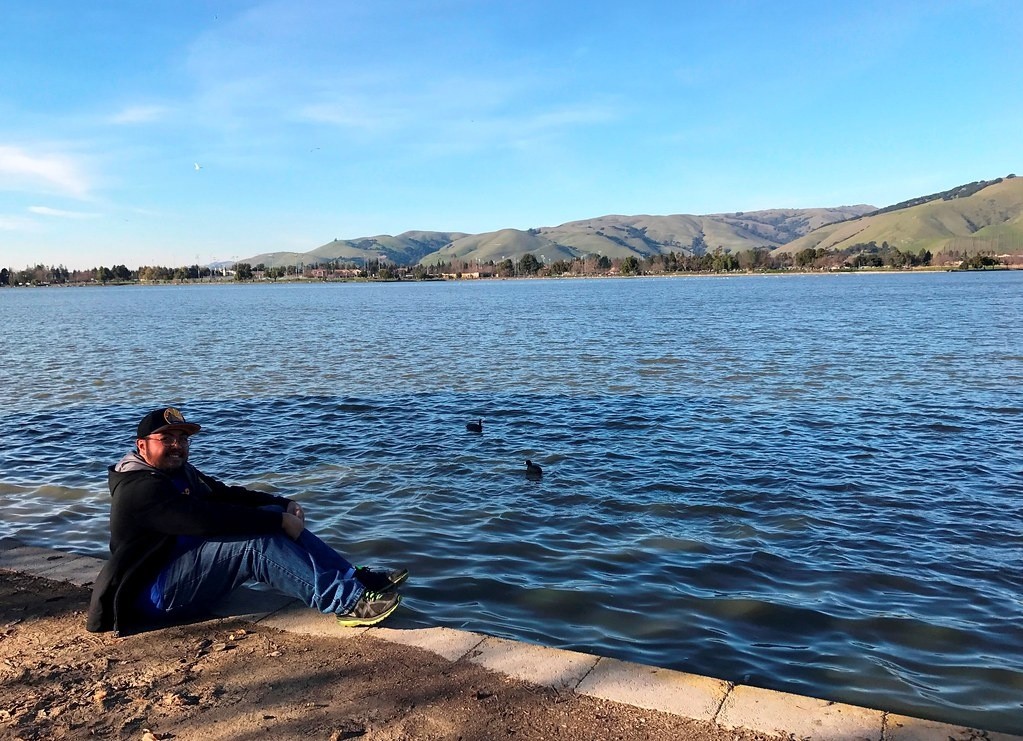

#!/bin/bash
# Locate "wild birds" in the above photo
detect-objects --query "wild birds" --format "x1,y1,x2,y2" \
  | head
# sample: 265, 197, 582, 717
466, 419, 483, 432
524, 459, 543, 481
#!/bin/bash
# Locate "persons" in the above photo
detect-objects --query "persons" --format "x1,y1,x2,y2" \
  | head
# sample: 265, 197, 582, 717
86, 408, 410, 638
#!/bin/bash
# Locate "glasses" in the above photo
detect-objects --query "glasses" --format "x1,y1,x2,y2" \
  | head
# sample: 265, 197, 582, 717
143, 437, 193, 447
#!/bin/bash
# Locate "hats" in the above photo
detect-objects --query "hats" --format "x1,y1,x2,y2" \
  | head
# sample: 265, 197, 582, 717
137, 407, 200, 442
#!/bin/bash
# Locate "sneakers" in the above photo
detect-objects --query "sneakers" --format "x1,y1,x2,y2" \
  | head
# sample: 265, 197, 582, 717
335, 590, 402, 627
351, 564, 409, 592
